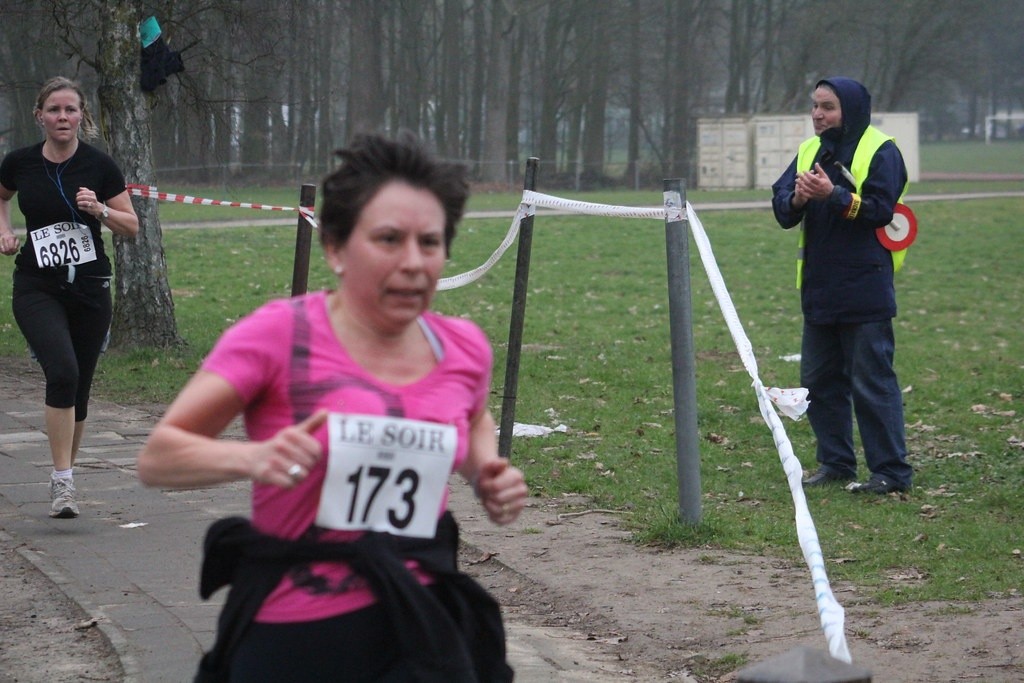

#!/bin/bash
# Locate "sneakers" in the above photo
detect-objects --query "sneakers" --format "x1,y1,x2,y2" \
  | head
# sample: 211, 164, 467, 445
46, 471, 79, 518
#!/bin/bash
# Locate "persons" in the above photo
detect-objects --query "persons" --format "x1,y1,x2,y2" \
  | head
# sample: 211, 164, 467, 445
771, 77, 911, 495
138, 128, 528, 683
0, 76, 140, 518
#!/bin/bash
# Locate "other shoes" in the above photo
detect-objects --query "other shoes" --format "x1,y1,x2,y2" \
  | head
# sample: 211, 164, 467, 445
801, 465, 857, 485
851, 476, 912, 495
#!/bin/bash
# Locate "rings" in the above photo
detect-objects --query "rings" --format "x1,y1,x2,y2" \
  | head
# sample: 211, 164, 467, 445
504, 503, 509, 515
288, 464, 303, 480
0, 246, 4, 249
88, 202, 92, 208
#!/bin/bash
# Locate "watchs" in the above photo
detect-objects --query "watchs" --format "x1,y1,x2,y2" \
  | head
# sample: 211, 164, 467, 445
96, 204, 108, 222
